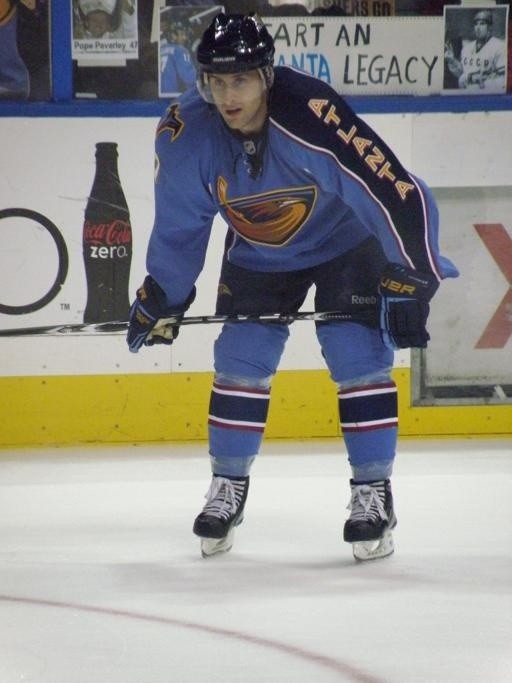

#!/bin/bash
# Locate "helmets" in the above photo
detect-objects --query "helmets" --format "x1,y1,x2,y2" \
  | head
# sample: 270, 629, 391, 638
196, 12, 276, 107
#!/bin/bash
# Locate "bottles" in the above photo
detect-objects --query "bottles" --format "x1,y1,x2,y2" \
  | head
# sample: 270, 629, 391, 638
79, 140, 136, 326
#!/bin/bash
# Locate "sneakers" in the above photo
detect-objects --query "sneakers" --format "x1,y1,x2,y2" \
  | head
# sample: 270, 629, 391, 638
192, 472, 251, 539
342, 476, 399, 544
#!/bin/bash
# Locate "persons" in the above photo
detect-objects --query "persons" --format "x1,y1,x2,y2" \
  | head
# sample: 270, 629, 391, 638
442, 9, 508, 94
160, 17, 199, 94
126, 10, 460, 542
73, 0, 132, 37
1, 2, 44, 103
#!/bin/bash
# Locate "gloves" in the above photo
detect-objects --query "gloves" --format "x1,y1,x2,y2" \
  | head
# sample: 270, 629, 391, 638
379, 261, 438, 353
126, 275, 197, 354
